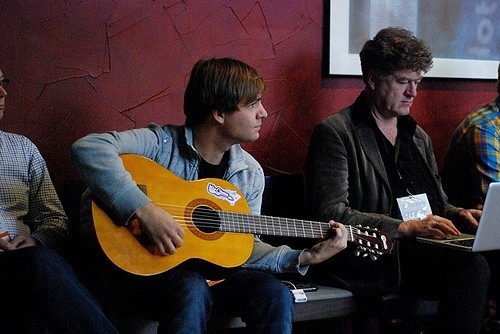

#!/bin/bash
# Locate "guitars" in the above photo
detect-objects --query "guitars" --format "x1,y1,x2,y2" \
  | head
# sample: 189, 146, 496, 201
80, 153, 393, 278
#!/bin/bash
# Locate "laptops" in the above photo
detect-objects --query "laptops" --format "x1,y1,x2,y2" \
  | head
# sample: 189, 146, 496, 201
416, 182, 500, 252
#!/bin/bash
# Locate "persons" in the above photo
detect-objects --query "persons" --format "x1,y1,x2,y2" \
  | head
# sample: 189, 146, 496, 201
0, 68, 120, 334
442, 62, 500, 334
68, 57, 348, 334
306, 27, 490, 334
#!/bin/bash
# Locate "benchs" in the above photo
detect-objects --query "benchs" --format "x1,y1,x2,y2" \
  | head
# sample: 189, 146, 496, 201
52, 173, 356, 334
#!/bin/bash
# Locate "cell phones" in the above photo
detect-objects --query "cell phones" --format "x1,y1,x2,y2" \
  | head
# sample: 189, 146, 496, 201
287, 283, 318, 293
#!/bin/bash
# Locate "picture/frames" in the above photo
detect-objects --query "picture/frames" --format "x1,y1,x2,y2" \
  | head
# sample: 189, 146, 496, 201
322, 0, 500, 83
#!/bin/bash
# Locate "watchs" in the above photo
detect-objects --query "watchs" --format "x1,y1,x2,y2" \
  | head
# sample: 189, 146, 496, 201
457, 208, 463, 213
